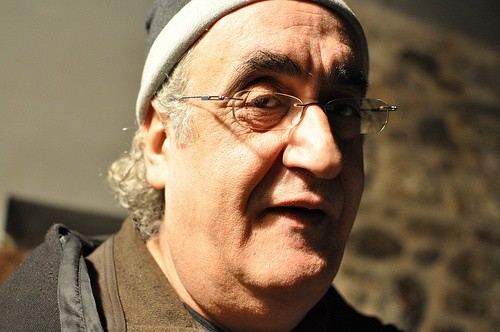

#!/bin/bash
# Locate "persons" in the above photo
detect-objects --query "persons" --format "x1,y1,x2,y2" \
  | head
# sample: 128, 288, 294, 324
0, 0, 407, 331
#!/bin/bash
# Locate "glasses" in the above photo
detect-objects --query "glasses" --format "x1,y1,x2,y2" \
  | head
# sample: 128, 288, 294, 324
169, 89, 397, 136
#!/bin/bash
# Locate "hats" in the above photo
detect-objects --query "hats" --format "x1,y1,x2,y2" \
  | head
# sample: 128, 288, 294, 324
135, 0, 370, 127
